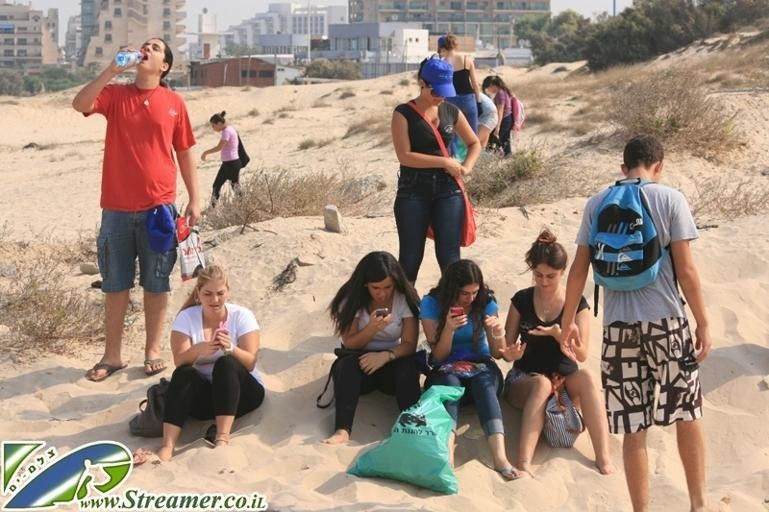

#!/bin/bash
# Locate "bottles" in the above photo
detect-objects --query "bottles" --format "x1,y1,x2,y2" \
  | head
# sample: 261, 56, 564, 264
116, 49, 143, 69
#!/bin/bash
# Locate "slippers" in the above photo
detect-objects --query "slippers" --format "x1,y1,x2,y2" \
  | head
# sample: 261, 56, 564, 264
143, 356, 168, 376
493, 462, 522, 481
85, 361, 128, 383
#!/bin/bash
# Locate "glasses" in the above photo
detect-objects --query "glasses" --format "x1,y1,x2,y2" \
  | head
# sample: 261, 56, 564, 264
425, 83, 445, 97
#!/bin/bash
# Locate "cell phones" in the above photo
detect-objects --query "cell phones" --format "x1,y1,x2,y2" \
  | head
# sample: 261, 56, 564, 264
451, 307, 464, 317
375, 307, 388, 318
213, 329, 229, 345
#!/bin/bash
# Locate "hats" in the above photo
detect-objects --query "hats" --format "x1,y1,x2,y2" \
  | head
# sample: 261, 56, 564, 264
147, 204, 177, 253
419, 58, 457, 98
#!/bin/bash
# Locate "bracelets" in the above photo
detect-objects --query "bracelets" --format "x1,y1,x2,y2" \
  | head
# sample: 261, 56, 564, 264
225, 342, 233, 354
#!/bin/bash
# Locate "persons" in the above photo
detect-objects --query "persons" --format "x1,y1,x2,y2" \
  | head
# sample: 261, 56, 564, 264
201, 110, 243, 214
156, 264, 265, 464
71, 39, 201, 381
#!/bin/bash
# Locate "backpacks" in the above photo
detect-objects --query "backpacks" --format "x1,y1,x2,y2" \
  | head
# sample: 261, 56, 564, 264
588, 177, 668, 293
509, 94, 527, 132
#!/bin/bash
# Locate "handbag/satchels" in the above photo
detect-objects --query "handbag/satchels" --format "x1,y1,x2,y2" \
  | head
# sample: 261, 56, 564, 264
234, 135, 250, 168
425, 182, 478, 249
437, 359, 488, 380
541, 372, 587, 450
333, 345, 396, 375
128, 377, 172, 438
174, 215, 207, 282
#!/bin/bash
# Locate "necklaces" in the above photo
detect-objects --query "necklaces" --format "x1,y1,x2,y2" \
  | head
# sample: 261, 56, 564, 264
134, 85, 158, 106
